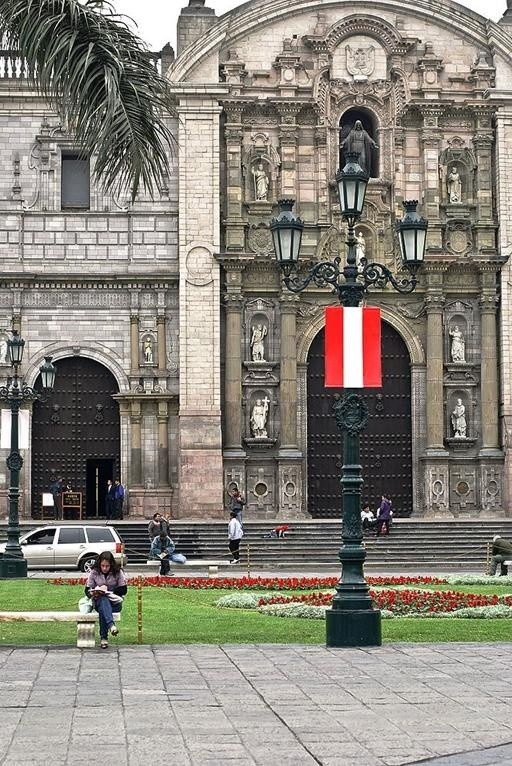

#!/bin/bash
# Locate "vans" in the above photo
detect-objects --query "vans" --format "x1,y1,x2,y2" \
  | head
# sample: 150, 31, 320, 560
1, 526, 127, 573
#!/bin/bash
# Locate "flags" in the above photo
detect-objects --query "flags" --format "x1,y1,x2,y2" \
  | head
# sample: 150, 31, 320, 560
325, 307, 381, 389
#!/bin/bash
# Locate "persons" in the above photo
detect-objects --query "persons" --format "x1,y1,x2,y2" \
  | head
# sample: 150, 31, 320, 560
263, 525, 288, 539
84, 551, 127, 649
353, 230, 366, 274
250, 324, 267, 362
485, 535, 512, 576
447, 167, 461, 202
251, 164, 270, 201
374, 494, 392, 537
143, 337, 152, 362
360, 505, 380, 532
105, 479, 116, 519
162, 513, 171, 540
449, 325, 466, 363
228, 488, 246, 527
149, 532, 175, 577
250, 400, 268, 438
49, 476, 63, 521
228, 512, 243, 564
112, 480, 124, 520
452, 398, 467, 439
147, 513, 161, 542
340, 120, 379, 178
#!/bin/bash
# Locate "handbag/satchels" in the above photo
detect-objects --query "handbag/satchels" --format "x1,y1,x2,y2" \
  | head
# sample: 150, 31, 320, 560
79, 596, 95, 614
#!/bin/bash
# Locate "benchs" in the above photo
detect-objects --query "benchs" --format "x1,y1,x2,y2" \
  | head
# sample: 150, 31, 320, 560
147, 559, 231, 578
0, 611, 121, 649
503, 560, 512, 566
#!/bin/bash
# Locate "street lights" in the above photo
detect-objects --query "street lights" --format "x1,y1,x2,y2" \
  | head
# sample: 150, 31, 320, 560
1, 330, 57, 577
266, 152, 428, 649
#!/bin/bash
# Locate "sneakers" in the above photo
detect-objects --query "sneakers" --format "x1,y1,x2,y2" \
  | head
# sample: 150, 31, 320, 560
165, 571, 174, 577
231, 558, 240, 564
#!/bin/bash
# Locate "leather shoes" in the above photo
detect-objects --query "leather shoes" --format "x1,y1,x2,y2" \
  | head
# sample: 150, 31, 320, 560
101, 642, 108, 649
110, 626, 119, 637
